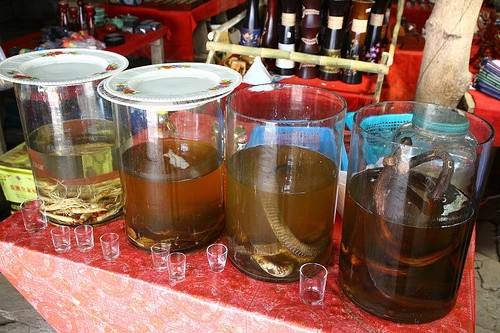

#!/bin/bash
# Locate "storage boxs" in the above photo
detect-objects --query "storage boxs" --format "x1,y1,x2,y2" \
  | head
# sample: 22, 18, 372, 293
0, 142, 37, 203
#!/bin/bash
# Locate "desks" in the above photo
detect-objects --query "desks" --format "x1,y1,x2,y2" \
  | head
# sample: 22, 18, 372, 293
0, 0, 500, 333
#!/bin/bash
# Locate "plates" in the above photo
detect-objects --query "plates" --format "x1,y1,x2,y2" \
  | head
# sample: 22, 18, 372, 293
0, 47, 130, 87
103, 62, 244, 102
97, 78, 234, 111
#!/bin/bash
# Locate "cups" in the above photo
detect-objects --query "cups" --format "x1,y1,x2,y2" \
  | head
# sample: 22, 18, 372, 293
74, 224, 94, 252
99, 232, 119, 260
150, 243, 170, 271
206, 242, 228, 272
299, 261, 328, 305
19, 198, 48, 230
50, 225, 71, 252
166, 252, 186, 280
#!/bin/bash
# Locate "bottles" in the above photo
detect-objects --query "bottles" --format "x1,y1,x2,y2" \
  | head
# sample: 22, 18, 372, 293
109, 101, 223, 255
0, 0, 126, 57
13, 83, 122, 228
338, 101, 494, 324
241, 0, 392, 86
223, 80, 349, 283
391, 106, 479, 194
